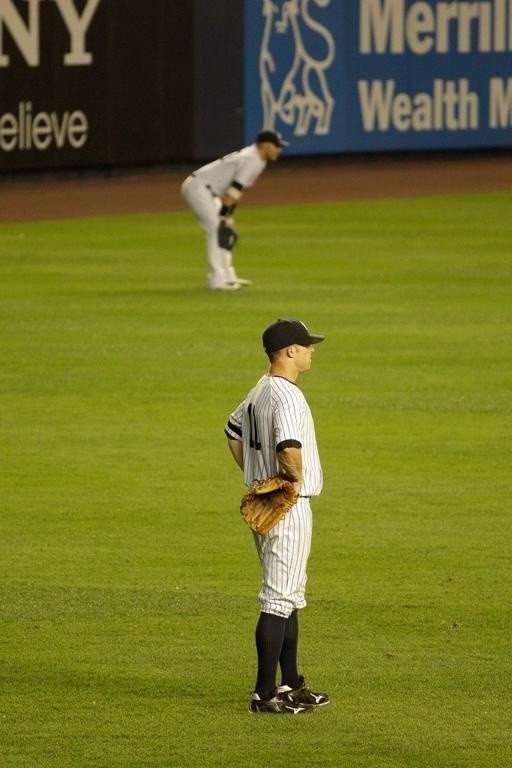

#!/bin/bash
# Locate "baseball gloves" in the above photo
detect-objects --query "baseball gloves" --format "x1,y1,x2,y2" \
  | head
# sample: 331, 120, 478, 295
240, 474, 303, 536
218, 220, 238, 251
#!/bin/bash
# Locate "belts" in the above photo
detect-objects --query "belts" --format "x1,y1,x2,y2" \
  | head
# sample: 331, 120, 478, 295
300, 495, 312, 500
190, 172, 217, 198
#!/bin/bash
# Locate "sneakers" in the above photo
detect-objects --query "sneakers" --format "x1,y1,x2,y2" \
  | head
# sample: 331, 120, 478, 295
217, 278, 251, 290
278, 675, 330, 707
248, 685, 314, 714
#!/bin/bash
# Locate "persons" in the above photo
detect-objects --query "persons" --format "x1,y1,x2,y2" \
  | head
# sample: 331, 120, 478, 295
225, 319, 331, 713
181, 129, 288, 290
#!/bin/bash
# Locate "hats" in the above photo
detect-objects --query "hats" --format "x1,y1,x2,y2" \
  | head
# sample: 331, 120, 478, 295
262, 319, 326, 353
256, 129, 290, 148
